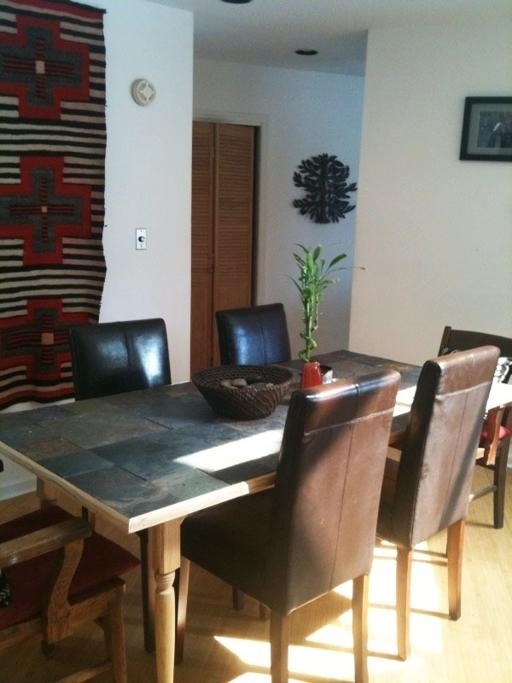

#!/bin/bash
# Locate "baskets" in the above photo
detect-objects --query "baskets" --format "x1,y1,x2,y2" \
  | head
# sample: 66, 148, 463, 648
191, 364, 294, 419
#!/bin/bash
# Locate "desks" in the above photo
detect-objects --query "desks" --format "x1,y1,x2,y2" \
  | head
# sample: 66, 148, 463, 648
0, 350, 512, 682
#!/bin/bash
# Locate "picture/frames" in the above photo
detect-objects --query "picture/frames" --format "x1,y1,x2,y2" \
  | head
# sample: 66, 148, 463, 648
458, 97, 512, 162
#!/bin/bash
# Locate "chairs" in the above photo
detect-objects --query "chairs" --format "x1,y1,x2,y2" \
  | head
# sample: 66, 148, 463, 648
383, 325, 512, 529
70, 319, 172, 401
376, 344, 501, 661
1, 458, 142, 682
173, 367, 401, 683
215, 303, 292, 366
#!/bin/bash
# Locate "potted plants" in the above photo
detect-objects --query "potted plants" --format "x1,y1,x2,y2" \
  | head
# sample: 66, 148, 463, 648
279, 242, 366, 389
